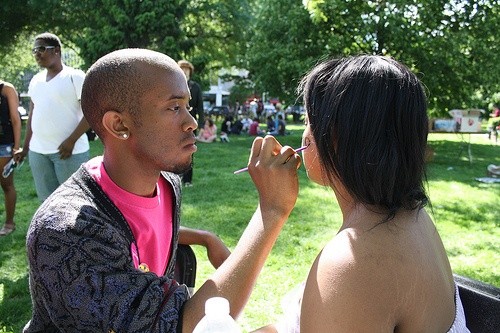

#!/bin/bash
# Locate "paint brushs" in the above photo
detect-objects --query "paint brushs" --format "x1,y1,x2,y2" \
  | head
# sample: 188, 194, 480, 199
233, 142, 311, 175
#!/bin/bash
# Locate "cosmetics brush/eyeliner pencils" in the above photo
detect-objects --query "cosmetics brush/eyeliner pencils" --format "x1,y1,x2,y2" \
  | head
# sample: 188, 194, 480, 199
234, 146, 307, 174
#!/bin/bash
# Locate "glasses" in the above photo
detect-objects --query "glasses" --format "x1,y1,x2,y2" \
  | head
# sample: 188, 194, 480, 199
31, 45, 55, 55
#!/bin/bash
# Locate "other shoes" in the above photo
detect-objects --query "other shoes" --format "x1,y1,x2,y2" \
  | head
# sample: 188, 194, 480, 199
0, 222, 16, 236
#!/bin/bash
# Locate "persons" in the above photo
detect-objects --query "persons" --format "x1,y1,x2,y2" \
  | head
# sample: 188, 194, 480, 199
21, 48, 301, 333
178, 59, 206, 187
199, 96, 285, 144
0, 33, 92, 235
248, 55, 470, 333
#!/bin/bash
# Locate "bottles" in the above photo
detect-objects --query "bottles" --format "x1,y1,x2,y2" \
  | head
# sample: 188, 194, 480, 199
190, 297, 243, 333
